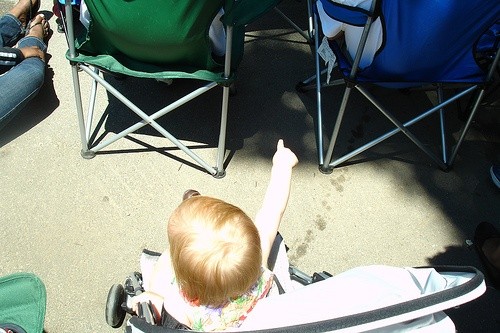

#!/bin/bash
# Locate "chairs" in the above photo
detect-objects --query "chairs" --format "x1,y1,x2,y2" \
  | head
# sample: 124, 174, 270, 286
297, 0, 500, 173
57, 0, 281, 178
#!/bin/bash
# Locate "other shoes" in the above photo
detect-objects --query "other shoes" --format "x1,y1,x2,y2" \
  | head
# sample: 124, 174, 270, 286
473, 221, 500, 291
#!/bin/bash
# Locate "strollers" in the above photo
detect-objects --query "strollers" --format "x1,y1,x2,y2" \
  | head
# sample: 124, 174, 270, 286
105, 265, 486, 333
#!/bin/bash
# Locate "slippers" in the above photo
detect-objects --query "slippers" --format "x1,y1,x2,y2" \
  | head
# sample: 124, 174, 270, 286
25, 14, 49, 42
16, 0, 40, 26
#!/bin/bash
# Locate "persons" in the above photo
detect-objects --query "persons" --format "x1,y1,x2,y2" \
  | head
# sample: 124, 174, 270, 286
0, 0, 54, 130
80, 0, 500, 291
161, 139, 298, 333
